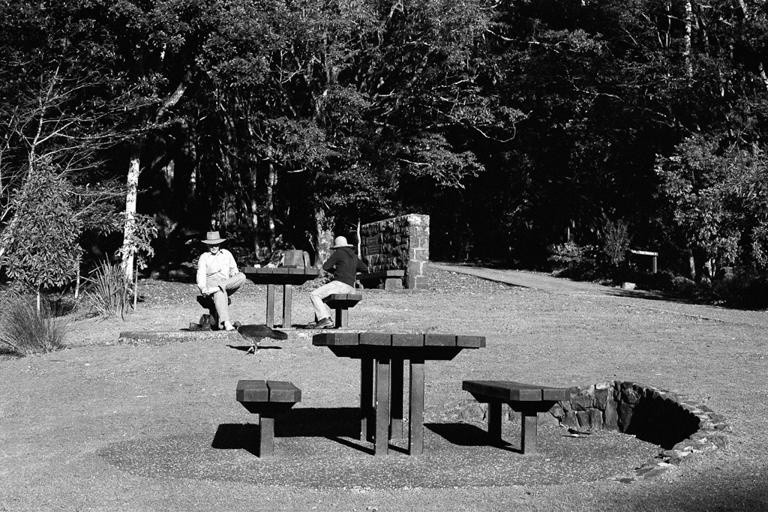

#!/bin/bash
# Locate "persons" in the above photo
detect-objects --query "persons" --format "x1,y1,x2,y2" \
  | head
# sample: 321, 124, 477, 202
309, 236, 368, 329
196, 231, 246, 331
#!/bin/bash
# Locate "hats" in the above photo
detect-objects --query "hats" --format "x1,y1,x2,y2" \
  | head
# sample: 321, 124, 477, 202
328, 235, 355, 249
200, 230, 227, 246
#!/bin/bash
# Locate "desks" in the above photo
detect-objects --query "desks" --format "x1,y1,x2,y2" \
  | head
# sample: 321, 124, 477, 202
244, 266, 319, 331
312, 332, 486, 454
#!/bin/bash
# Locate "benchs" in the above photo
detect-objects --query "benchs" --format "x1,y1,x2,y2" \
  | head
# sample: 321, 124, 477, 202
198, 294, 231, 330
323, 294, 364, 330
461, 379, 570, 454
237, 381, 301, 458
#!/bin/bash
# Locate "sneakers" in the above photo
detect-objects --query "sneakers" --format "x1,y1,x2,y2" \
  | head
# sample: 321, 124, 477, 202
305, 316, 336, 330
224, 321, 235, 330
